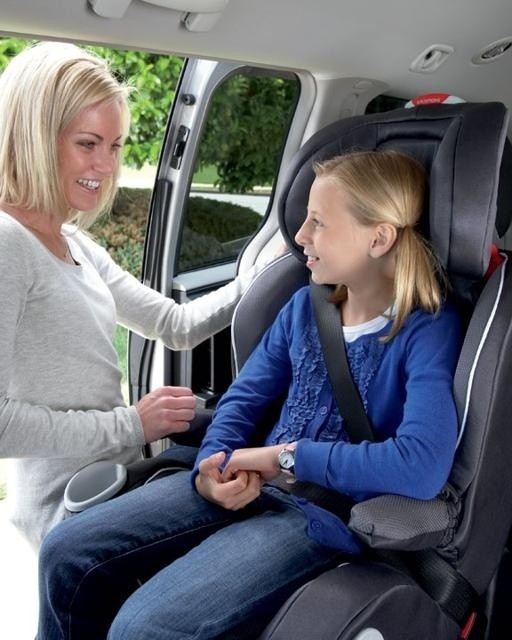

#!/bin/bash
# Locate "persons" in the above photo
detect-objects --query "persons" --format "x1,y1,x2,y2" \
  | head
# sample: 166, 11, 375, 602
1, 36, 299, 569
33, 145, 466, 639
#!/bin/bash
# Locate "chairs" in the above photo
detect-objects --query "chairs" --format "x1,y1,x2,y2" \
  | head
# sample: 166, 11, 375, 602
53, 102, 512, 639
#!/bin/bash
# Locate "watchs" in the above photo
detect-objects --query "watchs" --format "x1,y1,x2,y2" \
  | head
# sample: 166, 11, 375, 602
277, 444, 298, 477
